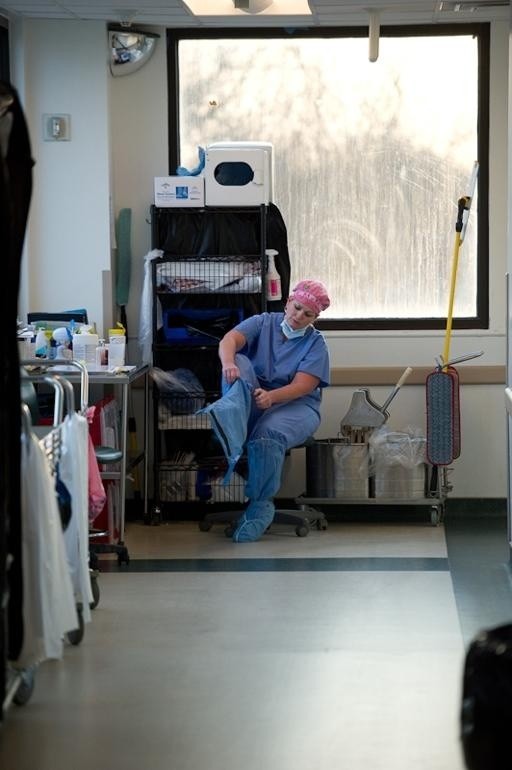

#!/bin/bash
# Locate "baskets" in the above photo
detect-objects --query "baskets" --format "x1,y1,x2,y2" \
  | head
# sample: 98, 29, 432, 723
157, 391, 222, 430
157, 463, 199, 502
156, 254, 262, 294
208, 466, 249, 503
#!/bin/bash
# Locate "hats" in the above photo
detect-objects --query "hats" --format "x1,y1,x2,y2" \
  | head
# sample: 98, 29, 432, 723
288, 280, 329, 316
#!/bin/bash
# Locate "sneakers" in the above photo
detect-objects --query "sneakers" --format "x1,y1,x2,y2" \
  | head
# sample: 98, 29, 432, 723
233, 500, 275, 542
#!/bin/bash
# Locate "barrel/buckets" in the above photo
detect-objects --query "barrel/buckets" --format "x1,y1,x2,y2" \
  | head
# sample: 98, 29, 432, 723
371, 439, 426, 500
305, 437, 369, 498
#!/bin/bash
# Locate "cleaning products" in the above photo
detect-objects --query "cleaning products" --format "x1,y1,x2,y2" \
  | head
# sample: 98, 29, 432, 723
35, 325, 48, 360
266, 247, 284, 302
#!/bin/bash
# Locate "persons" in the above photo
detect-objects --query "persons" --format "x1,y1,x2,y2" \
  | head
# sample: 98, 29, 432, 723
208, 281, 331, 544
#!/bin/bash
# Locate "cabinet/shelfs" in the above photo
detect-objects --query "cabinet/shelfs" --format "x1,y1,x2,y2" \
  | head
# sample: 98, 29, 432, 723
29, 364, 151, 552
151, 202, 271, 526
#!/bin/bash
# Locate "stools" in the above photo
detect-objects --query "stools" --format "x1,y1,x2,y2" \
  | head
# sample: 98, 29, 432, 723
198, 431, 329, 538
